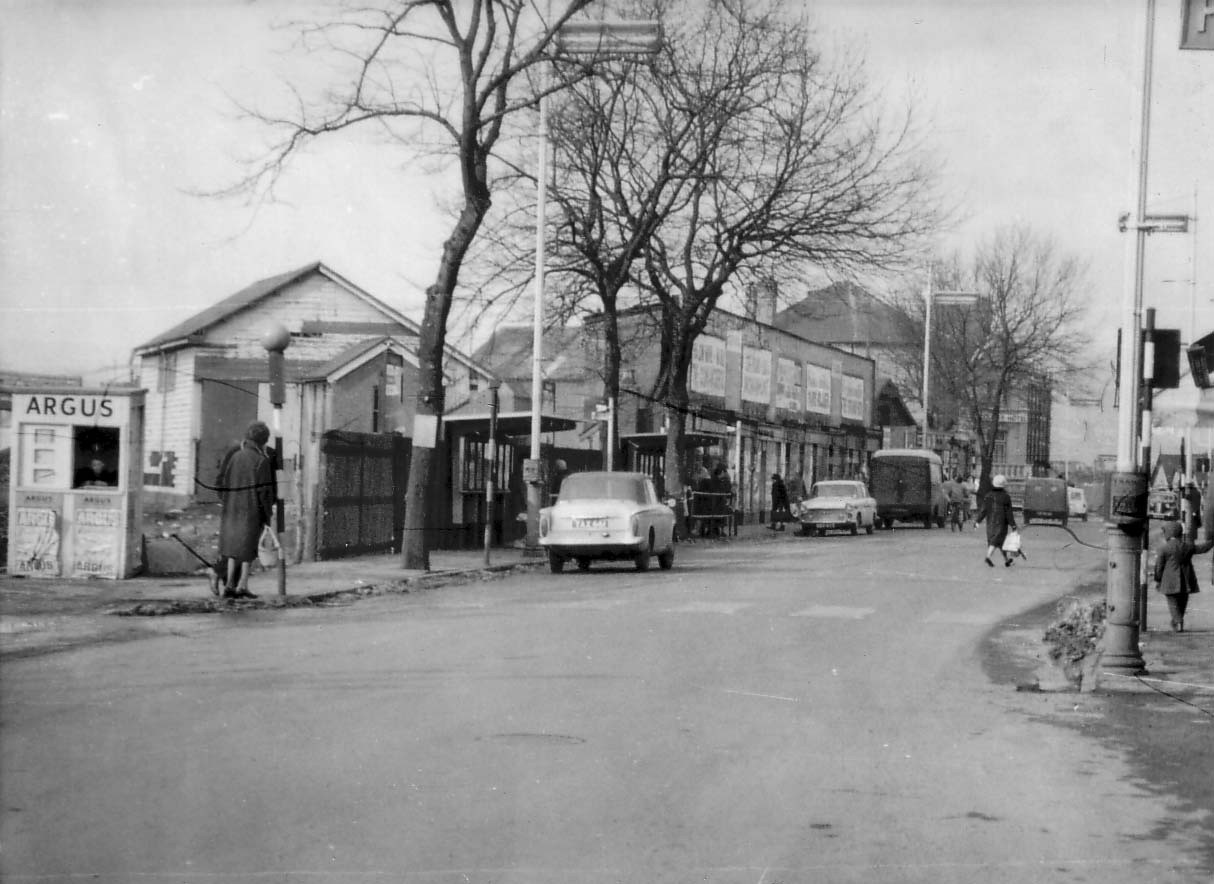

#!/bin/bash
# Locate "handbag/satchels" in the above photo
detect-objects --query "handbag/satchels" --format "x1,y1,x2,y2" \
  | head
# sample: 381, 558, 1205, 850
772, 505, 788, 521
255, 526, 281, 568
1002, 530, 1021, 553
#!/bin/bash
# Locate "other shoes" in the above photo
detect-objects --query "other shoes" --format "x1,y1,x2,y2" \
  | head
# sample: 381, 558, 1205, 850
1174, 623, 1182, 631
1005, 559, 1013, 567
986, 557, 993, 567
236, 587, 257, 599
223, 587, 236, 598
207, 568, 220, 597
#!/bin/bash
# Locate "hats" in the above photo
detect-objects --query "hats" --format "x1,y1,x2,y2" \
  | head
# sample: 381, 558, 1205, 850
246, 422, 270, 445
1161, 521, 1183, 541
993, 475, 1006, 488
771, 474, 781, 480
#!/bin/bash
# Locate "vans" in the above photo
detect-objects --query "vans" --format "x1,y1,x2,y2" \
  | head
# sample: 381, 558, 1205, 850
1069, 487, 1087, 521
870, 446, 951, 530
1023, 477, 1071, 526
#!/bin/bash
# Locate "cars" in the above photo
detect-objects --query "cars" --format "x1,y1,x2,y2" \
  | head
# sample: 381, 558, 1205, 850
800, 480, 878, 536
534, 470, 677, 570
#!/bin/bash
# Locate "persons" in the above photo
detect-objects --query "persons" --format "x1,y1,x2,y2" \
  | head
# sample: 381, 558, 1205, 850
205, 422, 277, 599
82, 456, 114, 486
766, 474, 791, 531
974, 474, 1016, 567
549, 459, 569, 506
1154, 521, 1214, 631
951, 477, 968, 521
668, 446, 731, 544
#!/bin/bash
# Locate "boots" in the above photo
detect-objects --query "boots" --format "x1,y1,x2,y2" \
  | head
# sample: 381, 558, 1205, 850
766, 523, 776, 529
776, 526, 785, 531
